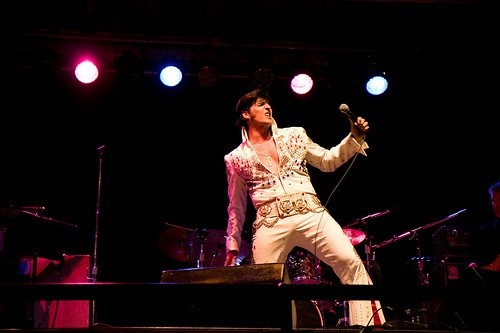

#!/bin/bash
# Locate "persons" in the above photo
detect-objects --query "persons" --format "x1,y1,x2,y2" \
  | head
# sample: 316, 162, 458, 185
223, 86, 388, 330
482, 182, 500, 273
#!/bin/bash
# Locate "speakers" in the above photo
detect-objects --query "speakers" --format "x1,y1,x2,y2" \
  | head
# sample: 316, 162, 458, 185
159, 263, 297, 328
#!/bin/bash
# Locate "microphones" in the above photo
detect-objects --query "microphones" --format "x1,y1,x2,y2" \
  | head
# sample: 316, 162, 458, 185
339, 104, 367, 134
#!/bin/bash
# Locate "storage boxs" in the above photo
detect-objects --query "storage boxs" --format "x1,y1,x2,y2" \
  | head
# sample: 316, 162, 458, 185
427, 224, 468, 252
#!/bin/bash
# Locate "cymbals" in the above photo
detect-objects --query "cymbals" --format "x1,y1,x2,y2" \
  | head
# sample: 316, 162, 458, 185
343, 227, 366, 246
22, 211, 77, 229
159, 228, 199, 261
195, 230, 250, 266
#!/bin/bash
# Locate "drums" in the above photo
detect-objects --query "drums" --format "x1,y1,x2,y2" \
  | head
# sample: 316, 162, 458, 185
286, 247, 322, 284
295, 299, 324, 328
407, 256, 432, 272
